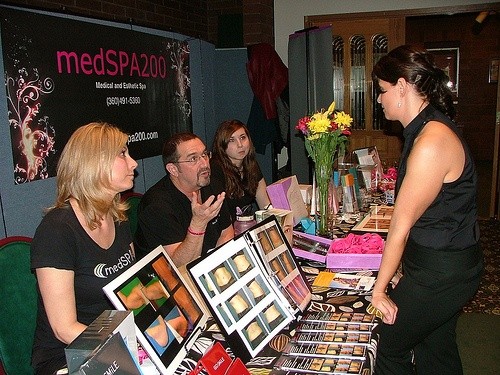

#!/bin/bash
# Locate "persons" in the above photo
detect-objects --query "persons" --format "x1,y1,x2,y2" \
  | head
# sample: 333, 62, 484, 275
137, 132, 234, 267
281, 212, 292, 244
332, 277, 358, 286
207, 119, 270, 225
368, 147, 383, 179
30, 121, 138, 375
371, 44, 485, 375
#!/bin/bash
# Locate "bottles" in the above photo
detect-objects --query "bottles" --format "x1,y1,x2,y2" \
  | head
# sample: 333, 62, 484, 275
234, 212, 257, 237
255, 210, 266, 224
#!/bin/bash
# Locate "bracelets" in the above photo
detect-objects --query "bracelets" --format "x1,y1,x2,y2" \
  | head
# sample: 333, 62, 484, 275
187, 227, 206, 235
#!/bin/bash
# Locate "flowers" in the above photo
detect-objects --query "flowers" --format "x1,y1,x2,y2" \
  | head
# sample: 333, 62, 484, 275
295, 101, 353, 233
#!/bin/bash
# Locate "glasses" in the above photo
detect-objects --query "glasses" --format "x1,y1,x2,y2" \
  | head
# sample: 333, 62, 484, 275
167, 151, 213, 164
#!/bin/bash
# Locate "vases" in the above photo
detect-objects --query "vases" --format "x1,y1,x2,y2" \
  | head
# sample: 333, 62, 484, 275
313, 171, 334, 237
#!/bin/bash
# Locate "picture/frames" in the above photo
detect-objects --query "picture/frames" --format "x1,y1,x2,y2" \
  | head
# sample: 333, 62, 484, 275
489, 56, 500, 84
426, 47, 460, 105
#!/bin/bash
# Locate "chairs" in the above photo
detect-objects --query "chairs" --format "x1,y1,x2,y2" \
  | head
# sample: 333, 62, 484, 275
0, 237, 40, 375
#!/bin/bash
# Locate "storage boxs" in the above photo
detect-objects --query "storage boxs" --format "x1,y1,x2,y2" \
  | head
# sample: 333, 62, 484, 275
266, 176, 385, 270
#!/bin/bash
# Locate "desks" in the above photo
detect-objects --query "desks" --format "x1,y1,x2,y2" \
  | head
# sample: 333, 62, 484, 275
246, 189, 404, 375
